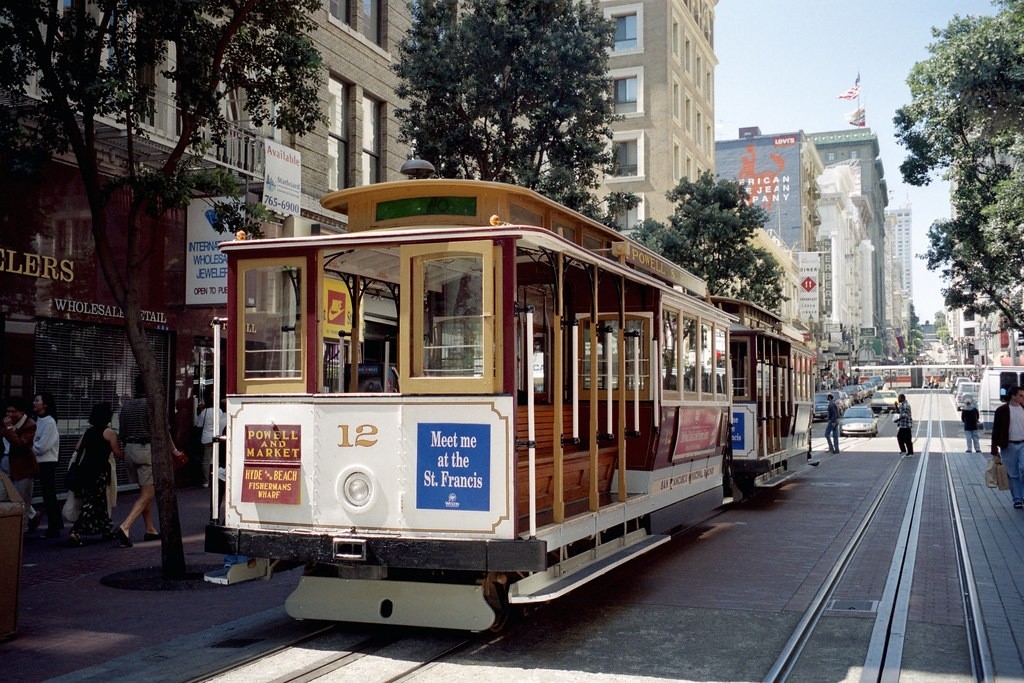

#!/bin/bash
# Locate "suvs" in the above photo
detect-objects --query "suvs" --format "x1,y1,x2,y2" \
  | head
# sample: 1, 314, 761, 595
954, 382, 980, 412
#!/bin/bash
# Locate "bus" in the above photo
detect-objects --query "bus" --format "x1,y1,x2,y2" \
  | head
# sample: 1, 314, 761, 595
694, 296, 820, 502
201, 160, 745, 635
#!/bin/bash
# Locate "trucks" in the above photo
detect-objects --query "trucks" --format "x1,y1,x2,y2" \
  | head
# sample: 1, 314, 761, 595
977, 366, 1024, 434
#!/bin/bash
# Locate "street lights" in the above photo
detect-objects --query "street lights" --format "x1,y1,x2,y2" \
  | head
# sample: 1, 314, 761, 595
960, 339, 970, 364
808, 313, 824, 392
863, 340, 873, 365
843, 324, 855, 385
977, 312, 993, 366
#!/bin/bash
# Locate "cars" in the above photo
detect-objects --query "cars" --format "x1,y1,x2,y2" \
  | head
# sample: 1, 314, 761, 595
870, 390, 902, 414
951, 377, 971, 395
839, 406, 879, 437
814, 376, 884, 419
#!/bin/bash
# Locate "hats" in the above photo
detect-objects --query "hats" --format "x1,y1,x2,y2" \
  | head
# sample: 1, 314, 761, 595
964, 398, 971, 405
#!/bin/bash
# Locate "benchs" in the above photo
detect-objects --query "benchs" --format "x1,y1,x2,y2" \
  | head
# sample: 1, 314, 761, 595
515, 405, 619, 519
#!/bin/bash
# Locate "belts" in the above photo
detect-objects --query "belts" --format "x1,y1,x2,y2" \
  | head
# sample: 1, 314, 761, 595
1008, 440, 1024, 444
125, 439, 152, 443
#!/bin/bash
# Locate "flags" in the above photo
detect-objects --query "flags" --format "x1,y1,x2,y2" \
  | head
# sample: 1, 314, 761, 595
850, 106, 866, 128
837, 78, 861, 100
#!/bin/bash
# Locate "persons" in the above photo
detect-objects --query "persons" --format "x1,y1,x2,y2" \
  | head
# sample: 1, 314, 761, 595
962, 398, 981, 452
825, 393, 839, 454
1, 373, 225, 547
894, 394, 915, 457
989, 386, 1024, 508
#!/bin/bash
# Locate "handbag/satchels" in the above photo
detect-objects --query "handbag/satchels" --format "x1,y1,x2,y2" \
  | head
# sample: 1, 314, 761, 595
985, 459, 997, 488
172, 449, 189, 472
61, 490, 84, 522
64, 463, 84, 492
997, 463, 1009, 490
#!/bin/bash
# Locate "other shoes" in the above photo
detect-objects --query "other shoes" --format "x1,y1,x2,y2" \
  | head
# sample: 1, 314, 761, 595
965, 450, 972, 453
69, 530, 83, 547
976, 450, 981, 452
114, 526, 133, 547
832, 449, 839, 453
40, 529, 61, 538
24, 511, 42, 533
904, 453, 914, 458
826, 448, 833, 452
202, 484, 209, 488
898, 452, 906, 455
144, 531, 161, 541
1014, 502, 1023, 508
